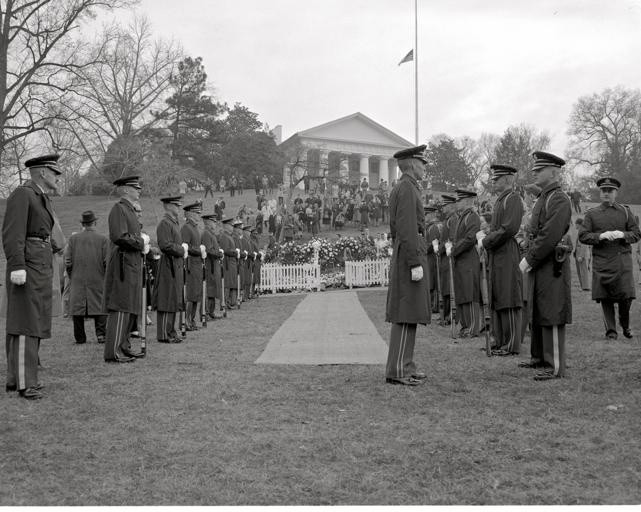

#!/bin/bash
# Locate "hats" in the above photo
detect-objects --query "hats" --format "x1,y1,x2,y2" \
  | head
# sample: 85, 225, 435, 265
79, 175, 260, 233
395, 145, 620, 214
25, 154, 62, 174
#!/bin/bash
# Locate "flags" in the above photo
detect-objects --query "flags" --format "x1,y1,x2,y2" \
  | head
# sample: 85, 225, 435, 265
399, 49, 414, 66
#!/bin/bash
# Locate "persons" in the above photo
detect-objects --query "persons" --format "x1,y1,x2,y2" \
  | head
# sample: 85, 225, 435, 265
419, 152, 576, 382
385, 144, 434, 385
2, 198, 70, 367
574, 218, 592, 292
261, 171, 429, 244
2, 156, 62, 401
572, 187, 583, 214
57, 174, 261, 364
578, 177, 641, 341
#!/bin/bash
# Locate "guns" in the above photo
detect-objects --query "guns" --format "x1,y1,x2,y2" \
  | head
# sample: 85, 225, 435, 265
139, 254, 147, 358
201, 258, 206, 326
237, 259, 241, 309
179, 259, 186, 336
220, 260, 226, 311
436, 253, 444, 326
481, 247, 491, 357
449, 255, 456, 338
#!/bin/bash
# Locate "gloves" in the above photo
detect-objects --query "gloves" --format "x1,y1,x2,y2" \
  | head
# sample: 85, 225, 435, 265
410, 228, 625, 282
10, 269, 26, 285
140, 233, 265, 261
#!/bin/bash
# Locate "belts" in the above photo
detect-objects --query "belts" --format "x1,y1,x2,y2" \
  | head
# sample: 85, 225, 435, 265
26, 234, 51, 243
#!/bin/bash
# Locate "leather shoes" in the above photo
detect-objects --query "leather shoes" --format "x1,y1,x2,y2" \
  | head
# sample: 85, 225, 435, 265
19, 387, 43, 400
97, 305, 238, 363
385, 316, 634, 386
6, 380, 45, 391
